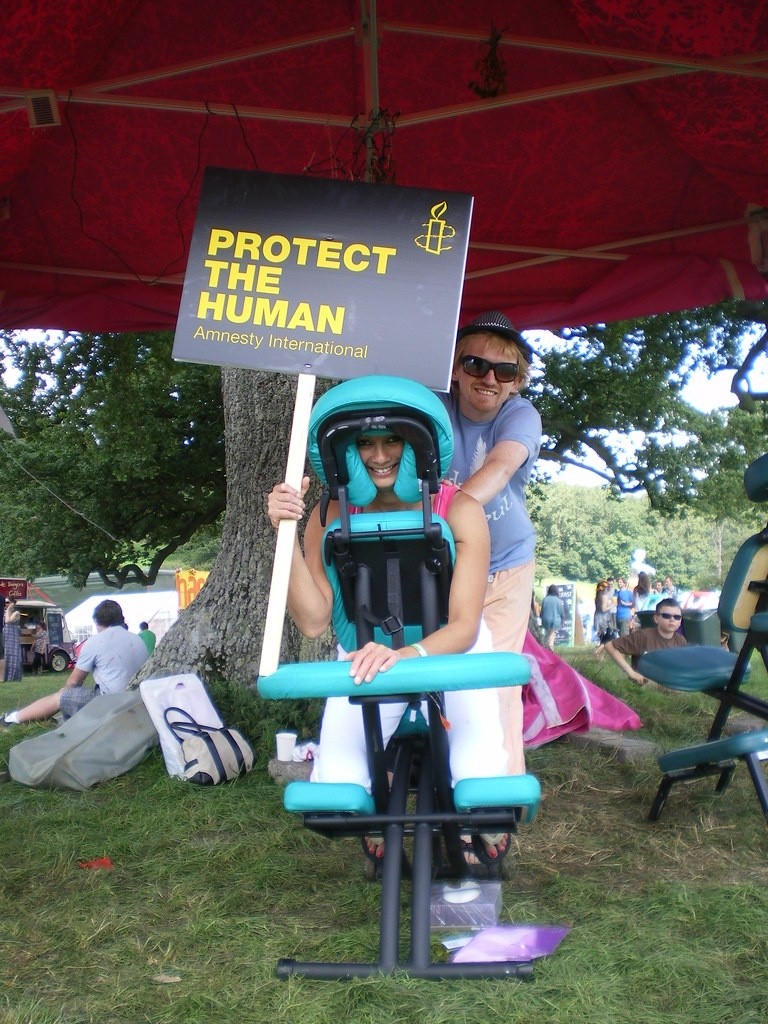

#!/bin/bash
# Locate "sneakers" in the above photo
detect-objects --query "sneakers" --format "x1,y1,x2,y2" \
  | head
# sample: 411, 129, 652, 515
0, 708, 21, 727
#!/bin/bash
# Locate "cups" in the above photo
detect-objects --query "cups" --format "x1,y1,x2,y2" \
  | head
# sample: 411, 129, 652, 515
275, 733, 297, 762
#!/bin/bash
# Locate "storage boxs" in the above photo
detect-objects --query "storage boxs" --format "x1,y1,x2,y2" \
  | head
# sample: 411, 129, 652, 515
20, 628, 35, 643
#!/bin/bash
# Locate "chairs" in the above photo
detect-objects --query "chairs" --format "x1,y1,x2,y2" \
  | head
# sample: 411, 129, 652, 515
632, 452, 768, 824
258, 373, 543, 985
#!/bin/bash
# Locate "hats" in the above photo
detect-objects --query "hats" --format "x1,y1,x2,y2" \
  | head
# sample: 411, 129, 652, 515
459, 312, 522, 339
595, 582, 609, 591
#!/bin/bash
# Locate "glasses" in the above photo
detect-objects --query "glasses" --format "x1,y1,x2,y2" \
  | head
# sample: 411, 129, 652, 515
5, 602, 9, 604
458, 355, 519, 383
659, 613, 682, 620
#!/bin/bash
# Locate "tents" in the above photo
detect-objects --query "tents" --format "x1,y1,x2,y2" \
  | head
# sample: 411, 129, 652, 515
62, 591, 179, 662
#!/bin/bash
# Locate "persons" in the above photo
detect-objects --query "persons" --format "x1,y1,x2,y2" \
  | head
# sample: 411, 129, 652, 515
30, 621, 48, 677
531, 585, 565, 650
594, 571, 681, 662
3, 596, 22, 682
137, 622, 156, 657
604, 598, 688, 684
0, 600, 150, 727
268, 434, 509, 789
364, 311, 543, 863
122, 623, 128, 630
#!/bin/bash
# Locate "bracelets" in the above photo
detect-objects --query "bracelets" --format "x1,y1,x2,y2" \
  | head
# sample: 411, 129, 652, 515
411, 644, 427, 657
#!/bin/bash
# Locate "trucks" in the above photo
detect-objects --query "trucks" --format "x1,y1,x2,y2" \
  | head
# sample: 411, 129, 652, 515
16, 601, 77, 673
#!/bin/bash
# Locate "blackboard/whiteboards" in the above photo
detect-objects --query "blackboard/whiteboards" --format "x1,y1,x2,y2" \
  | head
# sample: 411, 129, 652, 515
546, 584, 586, 647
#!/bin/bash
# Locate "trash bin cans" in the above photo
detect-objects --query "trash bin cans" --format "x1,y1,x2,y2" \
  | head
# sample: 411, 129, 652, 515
634, 608, 722, 647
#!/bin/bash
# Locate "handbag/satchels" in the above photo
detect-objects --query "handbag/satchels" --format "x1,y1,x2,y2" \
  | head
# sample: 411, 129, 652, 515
163, 707, 255, 785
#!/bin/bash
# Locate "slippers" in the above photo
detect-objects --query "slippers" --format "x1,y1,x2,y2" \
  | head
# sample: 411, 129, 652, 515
361, 836, 384, 863
471, 833, 511, 865
